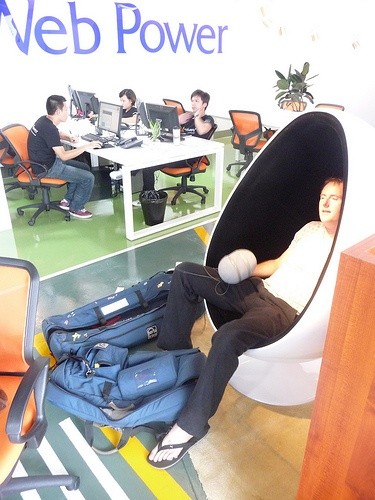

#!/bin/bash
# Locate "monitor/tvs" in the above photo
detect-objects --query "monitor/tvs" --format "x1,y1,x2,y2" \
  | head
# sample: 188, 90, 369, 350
69, 87, 123, 141
144, 102, 180, 133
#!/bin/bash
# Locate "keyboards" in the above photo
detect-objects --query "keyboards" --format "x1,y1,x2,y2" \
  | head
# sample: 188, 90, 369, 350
81, 132, 108, 142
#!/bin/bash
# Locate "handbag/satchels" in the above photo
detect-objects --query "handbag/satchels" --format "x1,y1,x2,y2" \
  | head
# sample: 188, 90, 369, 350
41, 271, 206, 348
45, 343, 208, 456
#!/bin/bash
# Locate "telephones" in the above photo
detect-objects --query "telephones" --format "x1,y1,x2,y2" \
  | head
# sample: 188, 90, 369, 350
119, 137, 143, 149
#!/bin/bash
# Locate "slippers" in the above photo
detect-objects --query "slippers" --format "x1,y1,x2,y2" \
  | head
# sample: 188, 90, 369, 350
146, 424, 210, 469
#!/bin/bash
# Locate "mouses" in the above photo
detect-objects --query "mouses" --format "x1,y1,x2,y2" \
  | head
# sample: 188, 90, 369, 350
94, 144, 103, 149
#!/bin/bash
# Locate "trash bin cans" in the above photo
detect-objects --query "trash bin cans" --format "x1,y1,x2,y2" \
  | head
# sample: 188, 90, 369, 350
140, 191, 168, 226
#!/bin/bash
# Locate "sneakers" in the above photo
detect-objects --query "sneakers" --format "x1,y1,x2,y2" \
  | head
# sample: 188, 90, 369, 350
68, 207, 94, 219
131, 200, 142, 207
58, 199, 70, 211
109, 168, 123, 180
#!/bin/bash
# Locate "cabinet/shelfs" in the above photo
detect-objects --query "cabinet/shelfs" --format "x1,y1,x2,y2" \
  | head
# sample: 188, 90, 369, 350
296, 233, 375, 500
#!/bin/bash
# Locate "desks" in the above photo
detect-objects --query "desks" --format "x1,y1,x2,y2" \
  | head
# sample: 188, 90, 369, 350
60, 127, 224, 241
234, 106, 301, 166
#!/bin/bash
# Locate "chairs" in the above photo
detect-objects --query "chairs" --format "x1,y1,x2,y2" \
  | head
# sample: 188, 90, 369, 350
159, 125, 219, 205
205, 111, 374, 406
0, 123, 71, 225
163, 99, 187, 114
0, 258, 79, 500
316, 103, 345, 111
264, 100, 307, 140
225, 110, 268, 177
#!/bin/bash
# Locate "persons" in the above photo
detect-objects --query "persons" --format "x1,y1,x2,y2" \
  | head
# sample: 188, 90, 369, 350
110, 89, 214, 207
146, 175, 345, 470
27, 95, 103, 219
119, 89, 140, 126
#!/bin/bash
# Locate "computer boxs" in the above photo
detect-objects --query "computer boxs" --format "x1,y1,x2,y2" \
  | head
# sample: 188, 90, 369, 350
119, 166, 144, 194
90, 166, 112, 201
